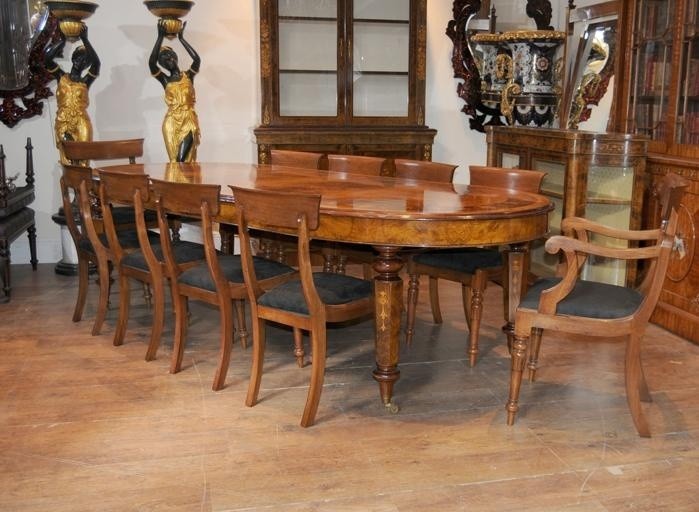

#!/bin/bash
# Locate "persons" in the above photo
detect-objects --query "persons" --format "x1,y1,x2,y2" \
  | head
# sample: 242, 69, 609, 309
148, 17, 201, 161
40, 18, 102, 210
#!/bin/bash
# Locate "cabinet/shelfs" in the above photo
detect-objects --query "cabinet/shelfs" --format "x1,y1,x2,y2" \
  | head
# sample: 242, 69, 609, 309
253, 124, 437, 272
257, 0, 431, 124
637, 161, 699, 347
617, 0, 699, 160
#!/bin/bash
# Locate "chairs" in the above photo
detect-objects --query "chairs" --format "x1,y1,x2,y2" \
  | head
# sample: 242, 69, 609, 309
229, 148, 325, 247
274, 153, 386, 273
406, 165, 546, 367
94, 165, 225, 362
147, 175, 298, 391
228, 183, 376, 430
503, 170, 688, 446
57, 160, 169, 337
56, 138, 174, 252
337, 159, 458, 280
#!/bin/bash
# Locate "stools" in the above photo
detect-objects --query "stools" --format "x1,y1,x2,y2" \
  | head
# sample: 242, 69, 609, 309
0, 209, 39, 305
0, 138, 38, 218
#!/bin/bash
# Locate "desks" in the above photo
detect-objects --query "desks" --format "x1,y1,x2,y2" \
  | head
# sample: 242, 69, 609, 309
94, 161, 553, 414
482, 123, 656, 285
